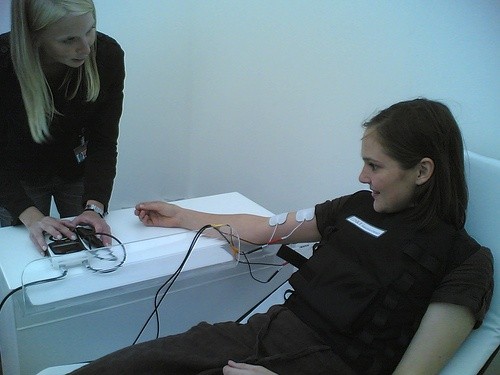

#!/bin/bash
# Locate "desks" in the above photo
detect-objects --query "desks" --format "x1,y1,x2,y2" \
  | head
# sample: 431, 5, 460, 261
0, 191, 298, 375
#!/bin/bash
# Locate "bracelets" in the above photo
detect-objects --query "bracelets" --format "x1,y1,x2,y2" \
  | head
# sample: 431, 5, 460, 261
84, 205, 104, 218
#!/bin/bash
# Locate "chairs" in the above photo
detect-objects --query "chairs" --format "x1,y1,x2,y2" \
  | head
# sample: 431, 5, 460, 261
36, 150, 500, 375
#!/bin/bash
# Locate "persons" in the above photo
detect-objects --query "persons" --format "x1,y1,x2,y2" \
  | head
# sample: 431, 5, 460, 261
0, 0, 126, 258
65, 98, 495, 375
